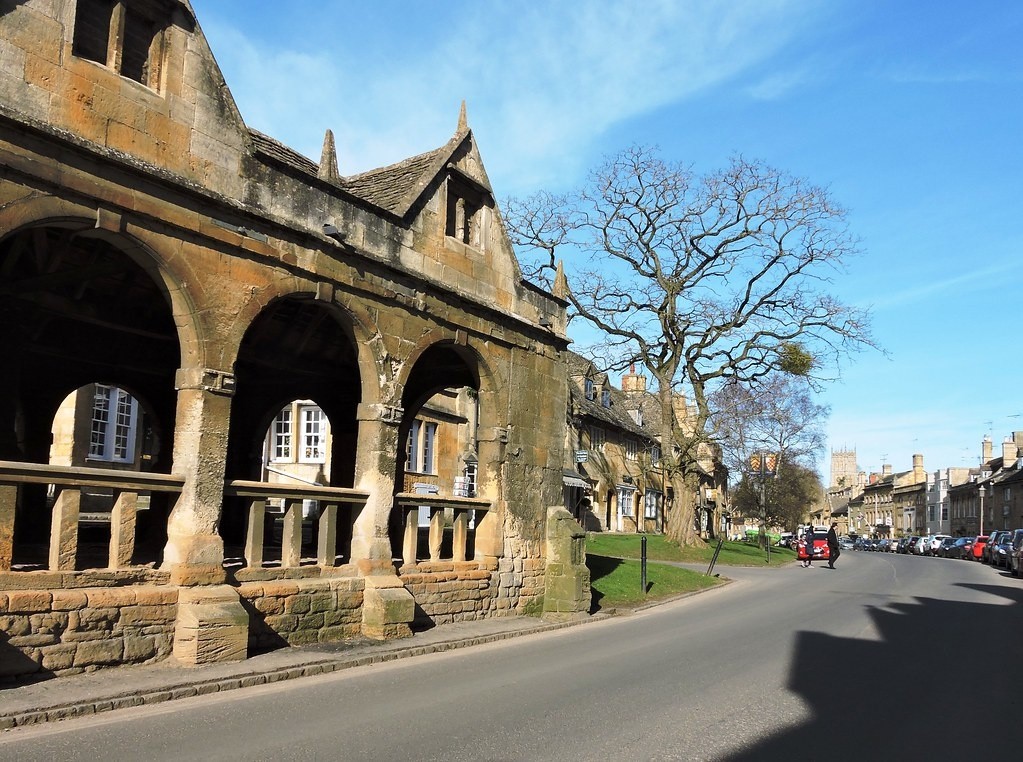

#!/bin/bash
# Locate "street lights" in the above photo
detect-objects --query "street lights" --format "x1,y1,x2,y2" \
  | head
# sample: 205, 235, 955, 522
749, 452, 776, 550
978, 485, 986, 536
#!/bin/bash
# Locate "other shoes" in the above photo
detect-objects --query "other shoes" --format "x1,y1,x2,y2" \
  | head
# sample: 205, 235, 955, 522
828, 561, 836, 569
808, 565, 815, 568
800, 561, 805, 567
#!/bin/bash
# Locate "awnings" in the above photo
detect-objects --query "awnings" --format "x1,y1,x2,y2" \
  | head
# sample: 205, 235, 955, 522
563, 476, 590, 508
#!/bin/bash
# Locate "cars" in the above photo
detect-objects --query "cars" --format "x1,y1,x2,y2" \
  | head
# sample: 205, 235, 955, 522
798, 532, 830, 559
839, 529, 1023, 579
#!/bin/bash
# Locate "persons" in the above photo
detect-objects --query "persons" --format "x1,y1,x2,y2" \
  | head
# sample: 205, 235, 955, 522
800, 526, 814, 568
827, 523, 840, 569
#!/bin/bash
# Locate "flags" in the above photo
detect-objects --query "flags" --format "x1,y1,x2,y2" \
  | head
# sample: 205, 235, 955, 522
855, 514, 864, 522
